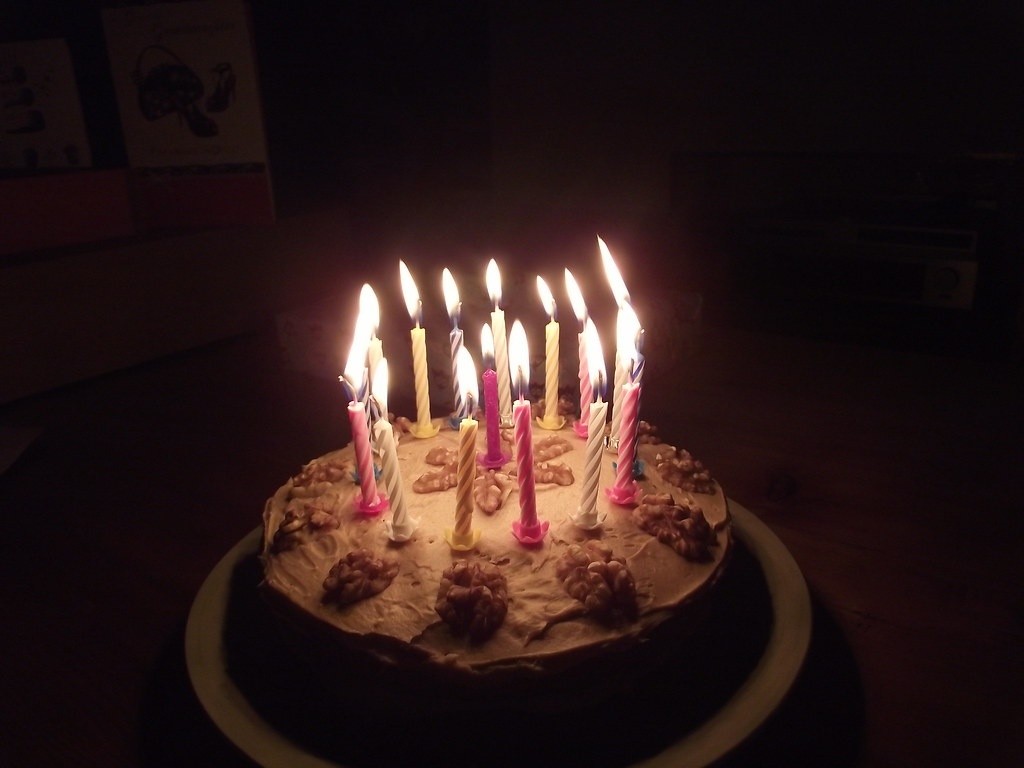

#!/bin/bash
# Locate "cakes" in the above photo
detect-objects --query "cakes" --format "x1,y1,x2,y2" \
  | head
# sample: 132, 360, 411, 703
260, 394, 732, 768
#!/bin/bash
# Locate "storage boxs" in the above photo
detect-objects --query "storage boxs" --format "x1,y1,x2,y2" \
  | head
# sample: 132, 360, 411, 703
0, 2, 276, 259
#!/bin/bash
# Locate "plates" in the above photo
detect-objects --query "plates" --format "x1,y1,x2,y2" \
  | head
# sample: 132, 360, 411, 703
183, 487, 811, 765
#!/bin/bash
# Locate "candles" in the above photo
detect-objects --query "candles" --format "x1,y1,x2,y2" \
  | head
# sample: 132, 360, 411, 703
338, 234, 647, 552
